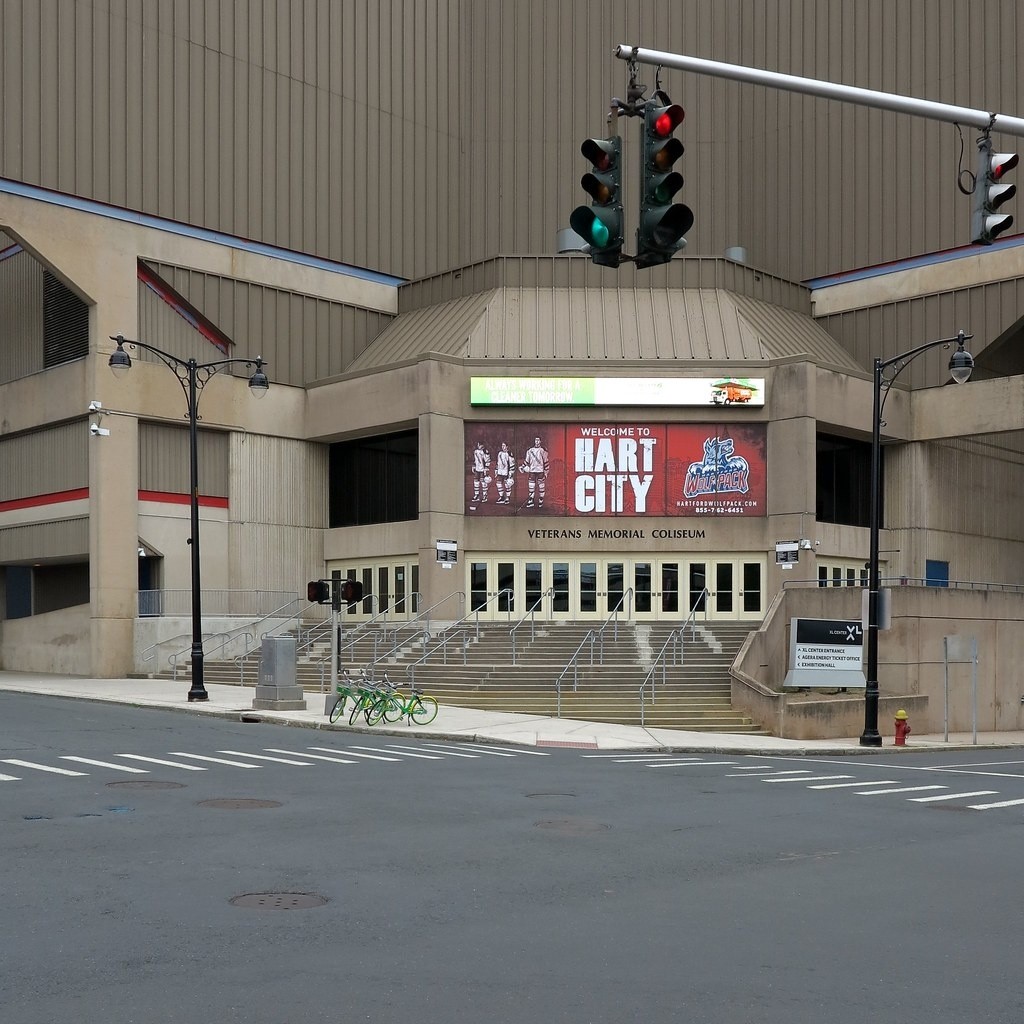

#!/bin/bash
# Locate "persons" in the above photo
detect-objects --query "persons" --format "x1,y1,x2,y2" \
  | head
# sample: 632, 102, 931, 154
471, 436, 491, 503
495, 443, 515, 504
518, 437, 549, 508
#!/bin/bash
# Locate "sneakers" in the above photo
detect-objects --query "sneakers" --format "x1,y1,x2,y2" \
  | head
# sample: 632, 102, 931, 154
471, 494, 480, 502
504, 498, 510, 504
496, 497, 505, 504
481, 495, 488, 503
538, 498, 543, 507
526, 497, 534, 508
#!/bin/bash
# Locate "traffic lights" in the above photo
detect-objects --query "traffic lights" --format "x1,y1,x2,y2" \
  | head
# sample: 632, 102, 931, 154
306, 581, 330, 603
969, 148, 1020, 245
635, 103, 694, 270
570, 134, 626, 271
340, 580, 363, 603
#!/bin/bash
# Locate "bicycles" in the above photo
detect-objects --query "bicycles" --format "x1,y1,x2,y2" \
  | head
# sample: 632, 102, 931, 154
349, 668, 406, 722
366, 668, 439, 727
329, 670, 384, 724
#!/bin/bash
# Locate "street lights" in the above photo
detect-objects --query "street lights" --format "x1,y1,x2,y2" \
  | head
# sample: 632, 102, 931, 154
108, 333, 270, 701
858, 328, 975, 747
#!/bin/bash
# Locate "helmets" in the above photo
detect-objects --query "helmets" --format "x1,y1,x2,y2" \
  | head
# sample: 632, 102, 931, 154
518, 465, 530, 473
484, 476, 492, 484
506, 478, 514, 488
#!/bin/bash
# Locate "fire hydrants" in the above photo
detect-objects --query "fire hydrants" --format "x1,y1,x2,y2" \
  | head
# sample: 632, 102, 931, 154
894, 708, 911, 747
